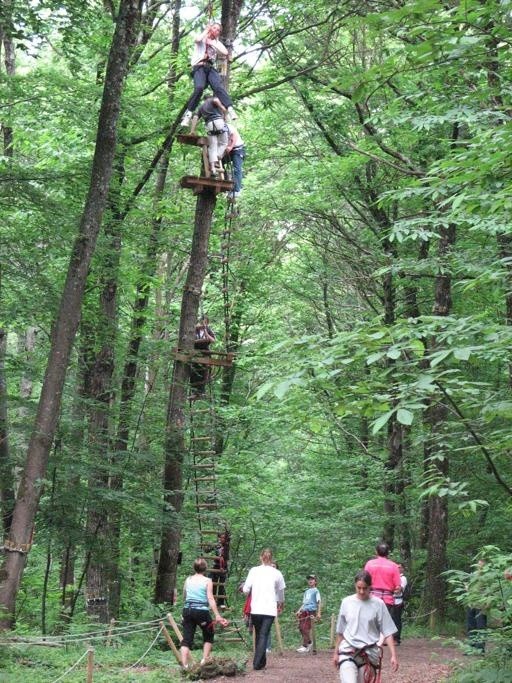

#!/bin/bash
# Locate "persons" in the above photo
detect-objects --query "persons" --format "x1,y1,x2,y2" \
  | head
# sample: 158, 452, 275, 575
224, 123, 246, 199
190, 315, 217, 398
391, 563, 407, 645
180, 21, 238, 127
265, 562, 278, 653
178, 557, 228, 678
295, 574, 322, 653
242, 548, 286, 670
463, 559, 491, 656
332, 570, 400, 683
363, 542, 402, 647
189, 93, 229, 179
202, 521, 231, 610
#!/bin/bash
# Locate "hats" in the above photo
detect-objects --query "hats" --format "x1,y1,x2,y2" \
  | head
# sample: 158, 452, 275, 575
306, 574, 317, 579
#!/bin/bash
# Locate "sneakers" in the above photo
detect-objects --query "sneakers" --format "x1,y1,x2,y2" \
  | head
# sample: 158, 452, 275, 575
394, 640, 400, 646
181, 113, 191, 127
182, 661, 196, 673
227, 108, 239, 121
266, 648, 272, 653
296, 639, 314, 652
201, 655, 216, 667
228, 191, 237, 198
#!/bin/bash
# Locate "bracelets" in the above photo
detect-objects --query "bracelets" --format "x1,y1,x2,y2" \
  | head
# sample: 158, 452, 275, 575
298, 610, 302, 612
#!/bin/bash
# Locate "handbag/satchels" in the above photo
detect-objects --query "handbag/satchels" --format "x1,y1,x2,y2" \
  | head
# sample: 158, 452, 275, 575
402, 583, 411, 601
